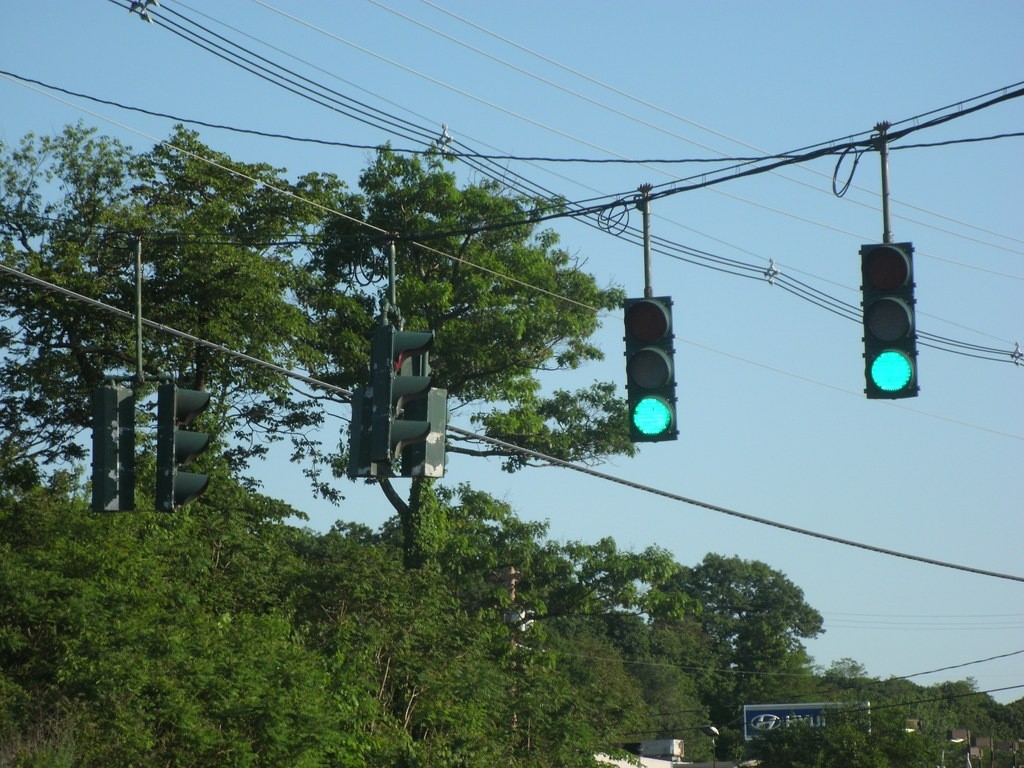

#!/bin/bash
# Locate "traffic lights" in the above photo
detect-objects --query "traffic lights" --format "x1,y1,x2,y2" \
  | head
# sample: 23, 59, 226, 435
369, 324, 432, 462
623, 296, 681, 444
860, 241, 921, 401
157, 383, 210, 513
